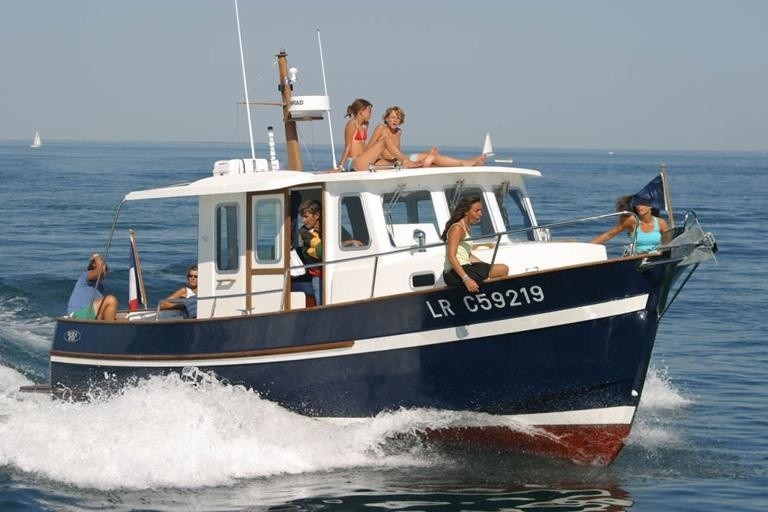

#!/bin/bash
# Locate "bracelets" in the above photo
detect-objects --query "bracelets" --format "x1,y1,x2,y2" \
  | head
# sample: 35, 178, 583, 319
462, 274, 470, 284
339, 162, 344, 169
93, 253, 99, 258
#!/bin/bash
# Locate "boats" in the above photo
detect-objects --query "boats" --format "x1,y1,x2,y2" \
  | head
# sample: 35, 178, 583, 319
19, 0, 719, 468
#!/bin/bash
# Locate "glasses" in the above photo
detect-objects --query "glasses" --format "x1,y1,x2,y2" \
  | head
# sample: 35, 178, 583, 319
188, 275, 198, 280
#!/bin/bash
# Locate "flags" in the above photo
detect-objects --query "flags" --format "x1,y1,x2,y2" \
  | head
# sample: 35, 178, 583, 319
128, 242, 144, 312
630, 175, 668, 209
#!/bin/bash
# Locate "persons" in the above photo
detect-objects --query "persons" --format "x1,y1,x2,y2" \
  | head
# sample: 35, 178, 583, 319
294, 200, 364, 307
589, 192, 670, 258
332, 98, 439, 174
366, 107, 488, 168
66, 253, 118, 322
161, 265, 198, 309
440, 192, 510, 293
